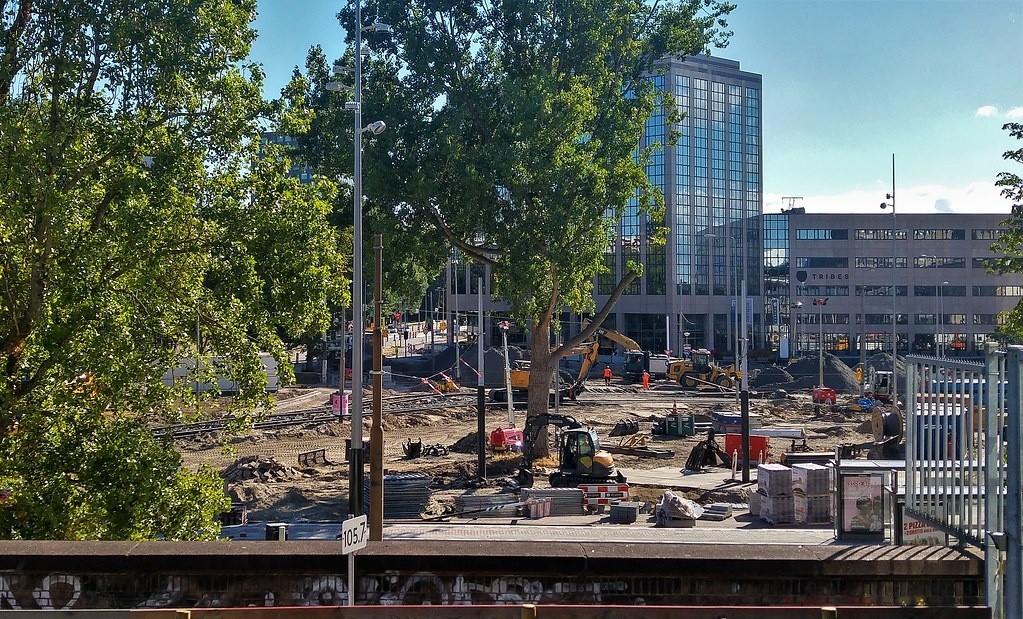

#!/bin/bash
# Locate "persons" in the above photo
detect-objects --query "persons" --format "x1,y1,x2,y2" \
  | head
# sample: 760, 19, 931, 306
604, 365, 612, 386
642, 370, 650, 391
851, 495, 882, 531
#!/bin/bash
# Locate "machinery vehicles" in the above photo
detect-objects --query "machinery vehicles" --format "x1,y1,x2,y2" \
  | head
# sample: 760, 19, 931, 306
488, 318, 643, 403
665, 347, 737, 390
621, 348, 670, 383
506, 412, 627, 489
863, 366, 904, 407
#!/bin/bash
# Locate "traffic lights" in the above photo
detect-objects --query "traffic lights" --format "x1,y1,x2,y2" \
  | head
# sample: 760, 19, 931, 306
404, 331, 408, 339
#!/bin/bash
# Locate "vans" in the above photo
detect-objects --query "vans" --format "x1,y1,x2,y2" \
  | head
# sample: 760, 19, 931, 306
490, 426, 524, 454
812, 387, 836, 403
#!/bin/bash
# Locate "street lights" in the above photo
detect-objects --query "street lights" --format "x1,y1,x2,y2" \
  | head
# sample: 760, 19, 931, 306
437, 287, 445, 339
812, 297, 830, 387
703, 234, 739, 370
324, 0, 395, 519
940, 282, 949, 358
920, 254, 939, 358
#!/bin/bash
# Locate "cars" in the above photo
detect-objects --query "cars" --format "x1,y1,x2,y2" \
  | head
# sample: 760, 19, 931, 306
387, 325, 397, 333
